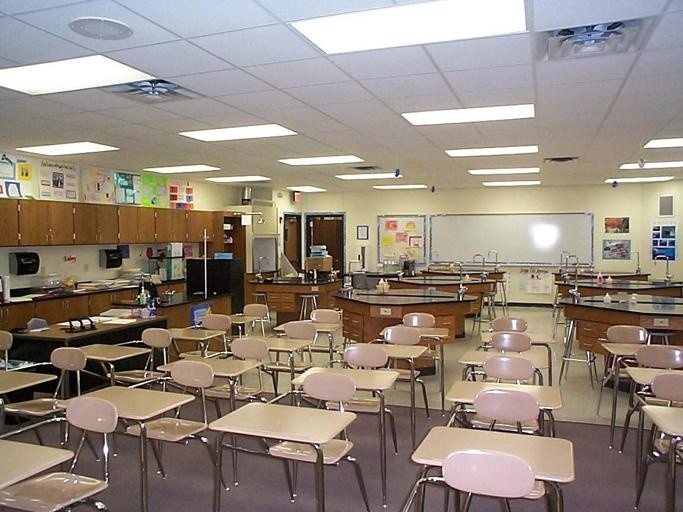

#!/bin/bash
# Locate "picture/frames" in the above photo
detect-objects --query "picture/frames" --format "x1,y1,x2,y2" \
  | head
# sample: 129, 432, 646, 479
357, 225, 368, 240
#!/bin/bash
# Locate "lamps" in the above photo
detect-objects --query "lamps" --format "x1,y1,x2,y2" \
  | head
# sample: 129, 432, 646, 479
246, 212, 267, 224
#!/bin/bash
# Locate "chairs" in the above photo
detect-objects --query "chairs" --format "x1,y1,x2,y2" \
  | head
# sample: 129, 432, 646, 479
492, 317, 527, 333
376, 327, 431, 418
393, 313, 440, 389
325, 345, 399, 455
303, 309, 345, 367
595, 326, 648, 415
634, 374, 683, 511
0, 331, 12, 369
179, 314, 233, 360
263, 322, 317, 397
202, 338, 268, 419
0, 396, 117, 512
484, 332, 531, 384
471, 357, 538, 435
107, 329, 181, 419
127, 361, 231, 491
231, 304, 272, 363
473, 390, 545, 500
3, 348, 100, 463
442, 450, 534, 512
270, 373, 371, 512
619, 346, 683, 454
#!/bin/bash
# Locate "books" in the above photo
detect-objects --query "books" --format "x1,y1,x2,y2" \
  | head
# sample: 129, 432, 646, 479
78, 268, 142, 290
310, 246, 328, 257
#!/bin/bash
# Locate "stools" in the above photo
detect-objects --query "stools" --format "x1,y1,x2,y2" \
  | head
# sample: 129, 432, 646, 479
252, 294, 271, 338
299, 295, 319, 321
552, 302, 569, 342
647, 332, 673, 346
491, 279, 509, 319
558, 318, 598, 390
472, 293, 496, 338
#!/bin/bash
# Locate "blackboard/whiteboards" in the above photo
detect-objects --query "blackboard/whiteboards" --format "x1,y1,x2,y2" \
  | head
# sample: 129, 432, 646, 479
429, 212, 594, 267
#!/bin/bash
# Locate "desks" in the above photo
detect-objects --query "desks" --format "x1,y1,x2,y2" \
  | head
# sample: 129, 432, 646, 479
380, 326, 449, 418
559, 294, 683, 393
420, 269, 506, 298
331, 289, 479, 376
599, 344, 683, 449
8, 315, 167, 397
343, 271, 404, 289
231, 315, 265, 340
248, 277, 343, 326
0, 439, 73, 493
274, 320, 342, 367
555, 278, 683, 297
445, 381, 562, 439
383, 276, 496, 317
77, 344, 151, 456
457, 346, 548, 386
150, 328, 227, 370
292, 368, 400, 508
366, 344, 426, 453
626, 367, 683, 486
111, 292, 233, 376
635, 406, 683, 512
552, 271, 651, 294
411, 426, 575, 512
209, 403, 356, 512
56, 385, 195, 512
481, 332, 555, 387
0, 370, 57, 435
244, 336, 314, 406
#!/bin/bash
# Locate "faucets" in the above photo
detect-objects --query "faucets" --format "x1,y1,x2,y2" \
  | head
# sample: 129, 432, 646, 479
655, 255, 672, 280
473, 254, 489, 283
453, 261, 468, 300
137, 274, 152, 300
630, 251, 641, 273
488, 249, 499, 272
341, 260, 363, 294
396, 255, 407, 280
565, 254, 581, 300
254, 256, 269, 281
559, 251, 570, 273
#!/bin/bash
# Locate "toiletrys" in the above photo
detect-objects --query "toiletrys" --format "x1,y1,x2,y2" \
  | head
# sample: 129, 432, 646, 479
629, 293, 637, 304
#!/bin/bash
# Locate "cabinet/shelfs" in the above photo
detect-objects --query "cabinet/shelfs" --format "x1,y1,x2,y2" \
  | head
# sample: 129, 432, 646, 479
0, 301, 35, 359
156, 280, 187, 297
187, 210, 213, 242
89, 287, 131, 317
19, 199, 73, 246
119, 205, 155, 244
75, 202, 118, 245
35, 292, 90, 325
207, 211, 246, 275
0, 198, 18, 247
156, 208, 186, 243
